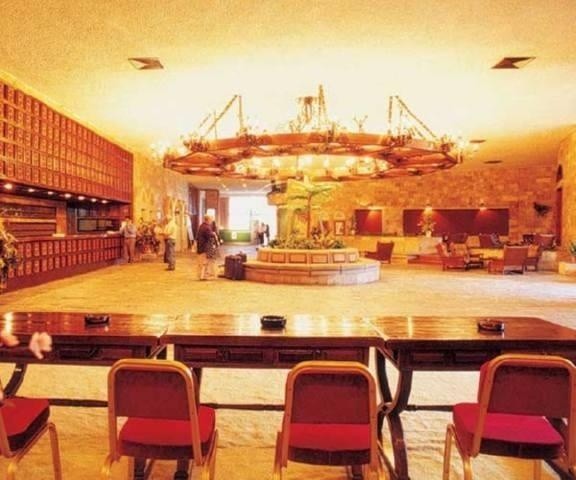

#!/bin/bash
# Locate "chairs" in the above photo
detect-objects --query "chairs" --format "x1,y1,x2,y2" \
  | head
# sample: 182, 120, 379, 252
271, 361, 386, 480
442, 354, 574, 479
365, 232, 562, 275
98, 359, 219, 479
0, 378, 61, 480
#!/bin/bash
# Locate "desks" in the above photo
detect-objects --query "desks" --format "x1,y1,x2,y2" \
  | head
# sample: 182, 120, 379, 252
369, 313, 574, 479
0, 311, 178, 425
154, 313, 385, 413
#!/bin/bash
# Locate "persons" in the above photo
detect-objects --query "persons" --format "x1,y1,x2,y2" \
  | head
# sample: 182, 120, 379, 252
479, 230, 503, 247
116, 212, 270, 282
442, 231, 452, 253
